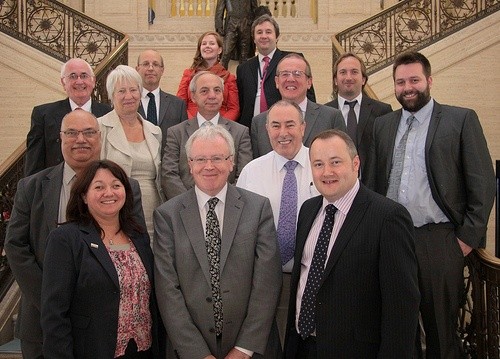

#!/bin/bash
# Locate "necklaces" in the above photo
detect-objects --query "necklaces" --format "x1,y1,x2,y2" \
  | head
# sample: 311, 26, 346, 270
106, 234, 114, 245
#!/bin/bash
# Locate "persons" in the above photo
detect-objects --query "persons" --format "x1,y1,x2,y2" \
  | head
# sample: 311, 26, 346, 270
237, 51, 498, 359
177, 32, 240, 121
215, 0, 258, 70
236, 13, 316, 136
4, 50, 285, 359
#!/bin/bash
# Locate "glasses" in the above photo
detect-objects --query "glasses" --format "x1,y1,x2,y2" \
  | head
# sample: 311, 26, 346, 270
191, 154, 233, 163
60, 130, 100, 136
64, 74, 89, 79
277, 70, 310, 78
139, 62, 163, 70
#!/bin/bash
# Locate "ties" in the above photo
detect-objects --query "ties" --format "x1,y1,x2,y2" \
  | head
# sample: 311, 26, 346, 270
147, 93, 157, 125
344, 99, 358, 152
204, 121, 212, 126
297, 204, 338, 341
275, 160, 299, 265
205, 197, 224, 336
386, 114, 415, 203
260, 56, 270, 113
